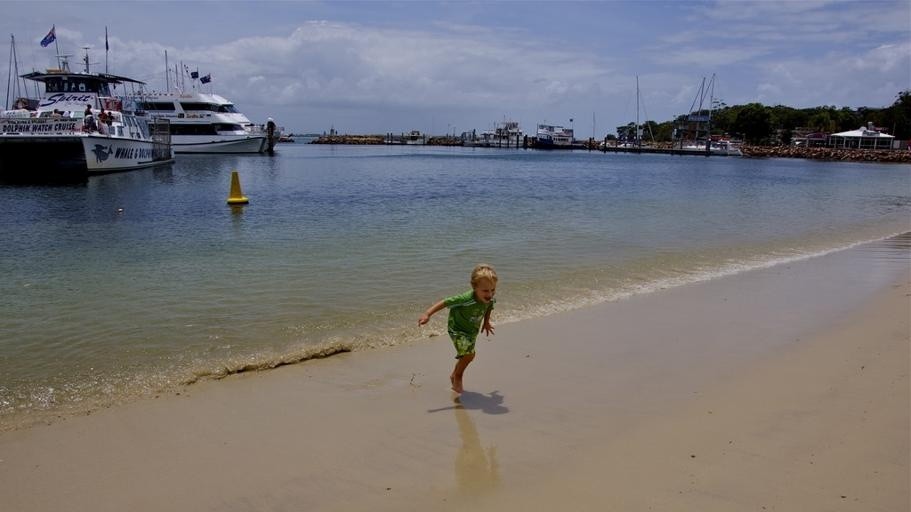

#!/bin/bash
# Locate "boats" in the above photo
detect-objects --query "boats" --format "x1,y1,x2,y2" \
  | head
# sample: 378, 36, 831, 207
116, 50, 281, 155
1, 22, 178, 178
279, 131, 296, 143
383, 129, 428, 144
461, 114, 527, 149
528, 121, 586, 150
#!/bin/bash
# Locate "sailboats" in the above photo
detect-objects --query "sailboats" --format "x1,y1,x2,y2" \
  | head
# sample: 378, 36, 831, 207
599, 72, 744, 157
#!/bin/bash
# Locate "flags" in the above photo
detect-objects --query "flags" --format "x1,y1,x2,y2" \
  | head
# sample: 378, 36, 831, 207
41, 27, 56, 48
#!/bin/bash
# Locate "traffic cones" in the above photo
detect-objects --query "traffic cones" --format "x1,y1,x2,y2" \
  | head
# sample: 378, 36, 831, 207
222, 172, 257, 208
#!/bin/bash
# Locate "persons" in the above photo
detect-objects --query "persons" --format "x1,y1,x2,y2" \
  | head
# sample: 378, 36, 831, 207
13, 97, 116, 135
417, 264, 497, 395
